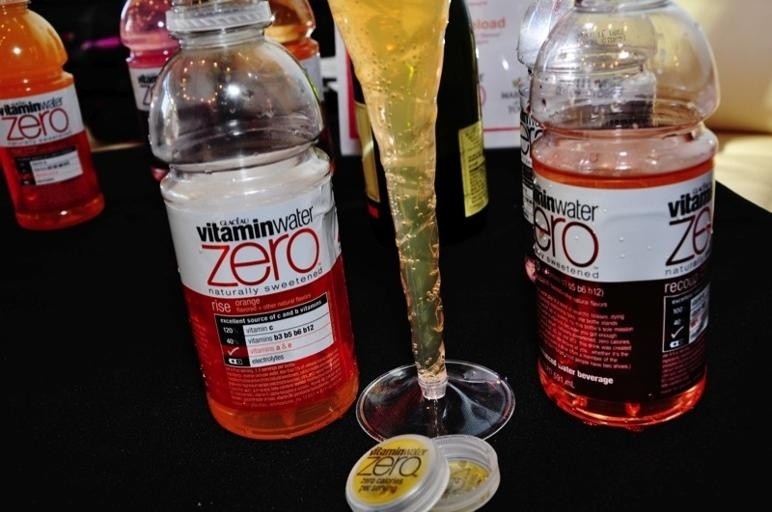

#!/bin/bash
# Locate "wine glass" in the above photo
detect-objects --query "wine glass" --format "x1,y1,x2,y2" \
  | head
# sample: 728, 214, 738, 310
329, 0, 515, 444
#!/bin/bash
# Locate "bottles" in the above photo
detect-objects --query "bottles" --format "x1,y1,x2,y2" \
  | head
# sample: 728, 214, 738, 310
344, 0, 496, 237
264, 0, 335, 186
1, 0, 104, 234
119, 0, 181, 182
517, 0, 656, 286
535, 1, 723, 434
148, 0, 359, 441
